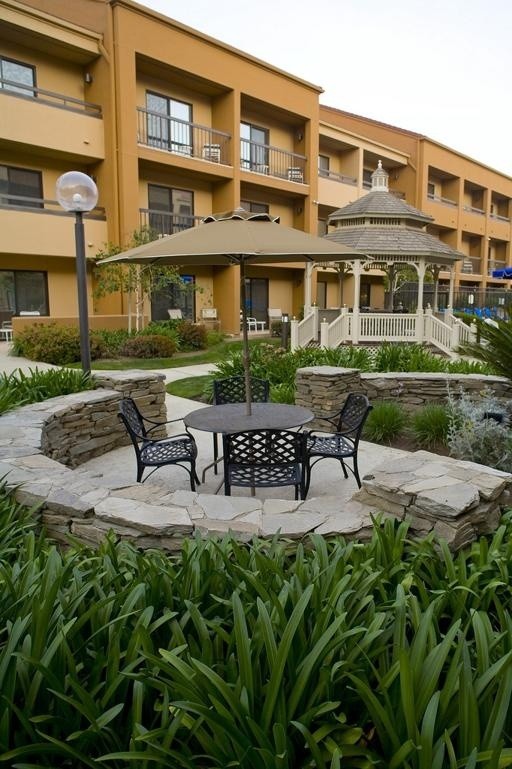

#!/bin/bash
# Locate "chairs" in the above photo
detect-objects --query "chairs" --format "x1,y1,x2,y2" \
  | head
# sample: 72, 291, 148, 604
288, 167, 303, 183
458, 305, 507, 321
299, 390, 372, 495
118, 397, 203, 495
1, 321, 13, 342
201, 142, 221, 164
210, 375, 270, 471
223, 428, 311, 502
244, 315, 266, 332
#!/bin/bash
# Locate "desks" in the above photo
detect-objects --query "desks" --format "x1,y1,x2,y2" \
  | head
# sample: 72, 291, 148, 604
183, 401, 313, 487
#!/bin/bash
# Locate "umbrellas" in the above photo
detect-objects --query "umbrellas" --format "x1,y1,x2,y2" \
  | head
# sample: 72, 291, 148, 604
492, 266, 512, 280
95, 206, 374, 496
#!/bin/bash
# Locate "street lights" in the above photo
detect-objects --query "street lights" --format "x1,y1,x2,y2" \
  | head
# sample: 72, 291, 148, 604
55, 170, 101, 378
